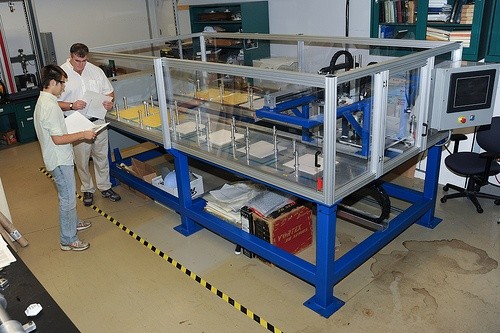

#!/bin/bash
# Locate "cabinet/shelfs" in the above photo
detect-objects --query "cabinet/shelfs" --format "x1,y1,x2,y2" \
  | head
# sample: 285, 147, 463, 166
0, 96, 38, 145
371, 0, 500, 66
189, 1, 270, 86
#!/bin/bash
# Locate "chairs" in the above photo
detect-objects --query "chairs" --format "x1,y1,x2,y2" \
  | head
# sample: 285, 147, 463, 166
441, 116, 500, 213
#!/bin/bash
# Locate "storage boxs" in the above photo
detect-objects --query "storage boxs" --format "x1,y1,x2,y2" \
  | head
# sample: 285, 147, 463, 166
151, 170, 204, 202
120, 158, 157, 194
241, 201, 311, 266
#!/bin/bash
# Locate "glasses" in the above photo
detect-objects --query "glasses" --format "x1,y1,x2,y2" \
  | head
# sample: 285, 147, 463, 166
56, 79, 65, 85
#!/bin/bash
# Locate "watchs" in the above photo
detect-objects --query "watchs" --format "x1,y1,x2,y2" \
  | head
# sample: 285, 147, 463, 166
69, 103, 73, 110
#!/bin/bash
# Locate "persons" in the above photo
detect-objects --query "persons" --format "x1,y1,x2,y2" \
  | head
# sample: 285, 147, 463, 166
33, 64, 97, 250
58, 42, 121, 206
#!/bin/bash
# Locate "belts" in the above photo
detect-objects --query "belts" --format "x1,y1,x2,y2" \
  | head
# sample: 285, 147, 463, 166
88, 117, 98, 123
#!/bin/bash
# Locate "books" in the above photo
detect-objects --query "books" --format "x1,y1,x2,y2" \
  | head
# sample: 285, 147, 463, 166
380, 0, 418, 24
378, 25, 409, 39
426, 0, 475, 47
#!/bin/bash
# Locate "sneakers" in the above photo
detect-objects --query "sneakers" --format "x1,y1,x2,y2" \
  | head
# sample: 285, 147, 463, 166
60, 239, 90, 251
83, 192, 93, 206
76, 218, 91, 231
102, 188, 121, 202
